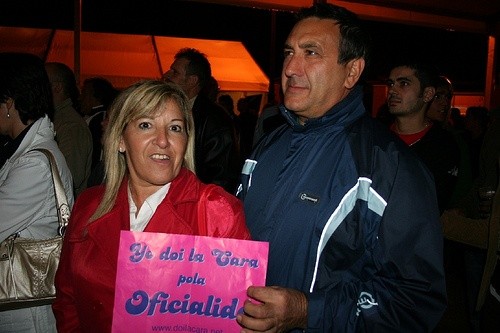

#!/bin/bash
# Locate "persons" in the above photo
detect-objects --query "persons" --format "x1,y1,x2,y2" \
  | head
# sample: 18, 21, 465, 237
381, 60, 500, 225
0, 46, 254, 333
235, 2, 450, 333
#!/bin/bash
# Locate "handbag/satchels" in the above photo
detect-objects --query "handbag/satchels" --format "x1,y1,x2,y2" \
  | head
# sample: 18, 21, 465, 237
0, 148, 72, 305
